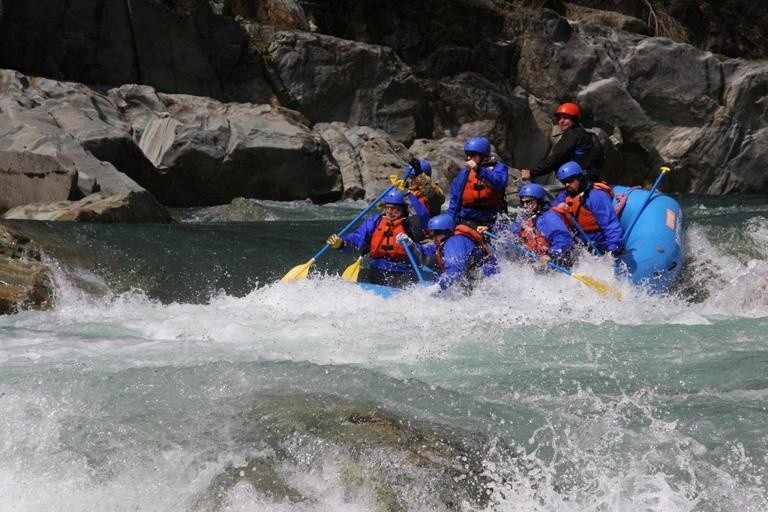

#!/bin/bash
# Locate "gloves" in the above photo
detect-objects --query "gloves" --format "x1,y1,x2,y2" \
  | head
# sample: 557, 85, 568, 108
420, 282, 442, 297
395, 232, 413, 247
408, 157, 424, 178
326, 233, 343, 250
389, 174, 406, 192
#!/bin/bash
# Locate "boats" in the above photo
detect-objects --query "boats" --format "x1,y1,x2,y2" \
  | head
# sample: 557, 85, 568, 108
327, 185, 687, 305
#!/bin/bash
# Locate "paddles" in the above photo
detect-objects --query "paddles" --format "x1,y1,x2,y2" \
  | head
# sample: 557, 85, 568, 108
484, 231, 622, 301
342, 166, 413, 282
281, 185, 393, 281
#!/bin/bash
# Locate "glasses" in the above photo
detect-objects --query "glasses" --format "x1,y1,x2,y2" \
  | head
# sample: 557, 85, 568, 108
521, 199, 536, 205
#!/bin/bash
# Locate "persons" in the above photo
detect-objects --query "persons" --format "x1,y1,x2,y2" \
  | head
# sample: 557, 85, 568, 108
519, 102, 604, 182
330, 188, 429, 272
476, 182, 576, 275
391, 155, 444, 216
552, 161, 625, 258
394, 213, 502, 296
446, 136, 509, 227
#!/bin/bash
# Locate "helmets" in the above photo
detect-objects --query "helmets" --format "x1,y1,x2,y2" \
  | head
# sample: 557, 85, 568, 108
405, 159, 432, 179
463, 136, 491, 157
555, 161, 586, 182
427, 213, 455, 232
554, 102, 582, 121
384, 187, 406, 205
518, 183, 545, 203
